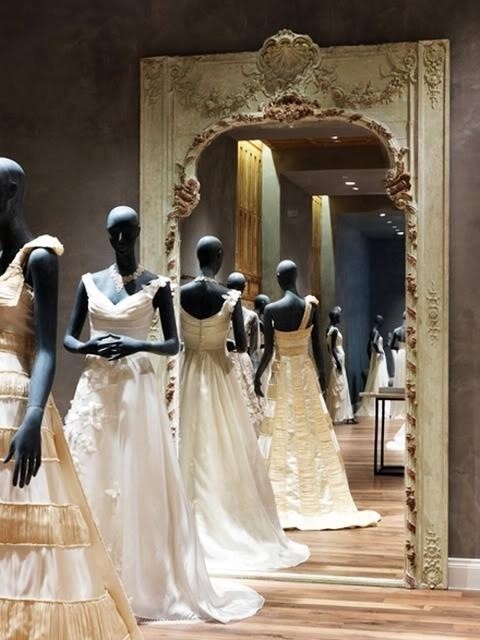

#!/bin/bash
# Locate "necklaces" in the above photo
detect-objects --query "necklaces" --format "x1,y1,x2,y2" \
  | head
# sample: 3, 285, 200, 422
107, 263, 148, 295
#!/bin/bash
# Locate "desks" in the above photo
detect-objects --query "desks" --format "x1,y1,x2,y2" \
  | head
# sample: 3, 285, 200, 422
356, 391, 405, 477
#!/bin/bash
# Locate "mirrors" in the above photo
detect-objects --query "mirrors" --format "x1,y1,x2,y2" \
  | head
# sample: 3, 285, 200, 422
164, 93, 421, 590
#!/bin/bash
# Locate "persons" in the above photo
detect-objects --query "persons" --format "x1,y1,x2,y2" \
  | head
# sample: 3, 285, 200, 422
253, 257, 381, 530
358, 312, 407, 420
323, 305, 358, 424
1, 158, 144, 640
60, 204, 266, 627
174, 233, 310, 574
